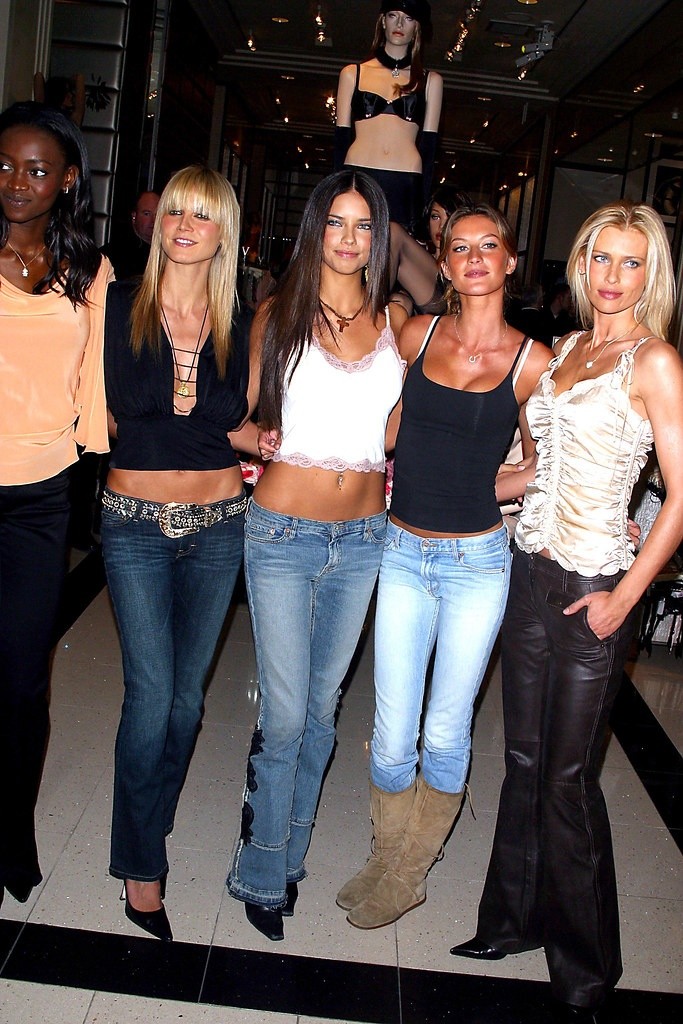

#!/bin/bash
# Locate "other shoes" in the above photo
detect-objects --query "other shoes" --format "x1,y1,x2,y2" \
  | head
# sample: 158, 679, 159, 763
3, 871, 34, 903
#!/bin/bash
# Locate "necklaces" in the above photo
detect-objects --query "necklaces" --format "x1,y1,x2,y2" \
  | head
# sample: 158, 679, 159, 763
5, 239, 46, 278
317, 294, 363, 333
159, 302, 209, 398
454, 314, 508, 362
586, 323, 636, 368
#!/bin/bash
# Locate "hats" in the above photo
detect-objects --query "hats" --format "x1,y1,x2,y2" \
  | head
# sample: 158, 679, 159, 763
378, 1, 432, 21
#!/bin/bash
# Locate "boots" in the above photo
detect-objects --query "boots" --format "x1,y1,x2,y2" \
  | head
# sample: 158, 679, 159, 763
334, 778, 419, 912
346, 776, 478, 931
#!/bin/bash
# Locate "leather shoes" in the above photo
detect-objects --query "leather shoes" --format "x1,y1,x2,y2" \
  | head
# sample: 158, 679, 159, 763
118, 875, 175, 944
244, 900, 285, 941
450, 935, 507, 961
281, 882, 299, 917
567, 1004, 603, 1024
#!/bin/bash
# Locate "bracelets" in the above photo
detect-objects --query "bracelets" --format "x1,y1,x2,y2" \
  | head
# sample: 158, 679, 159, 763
257, 438, 263, 456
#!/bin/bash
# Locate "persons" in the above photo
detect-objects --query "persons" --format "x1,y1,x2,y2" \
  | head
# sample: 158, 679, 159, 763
116, 166, 640, 943
333, 0, 475, 319
449, 197, 683, 1024
0, 102, 116, 903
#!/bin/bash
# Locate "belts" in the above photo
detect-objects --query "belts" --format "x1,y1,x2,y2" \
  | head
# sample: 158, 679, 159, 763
101, 485, 248, 540
